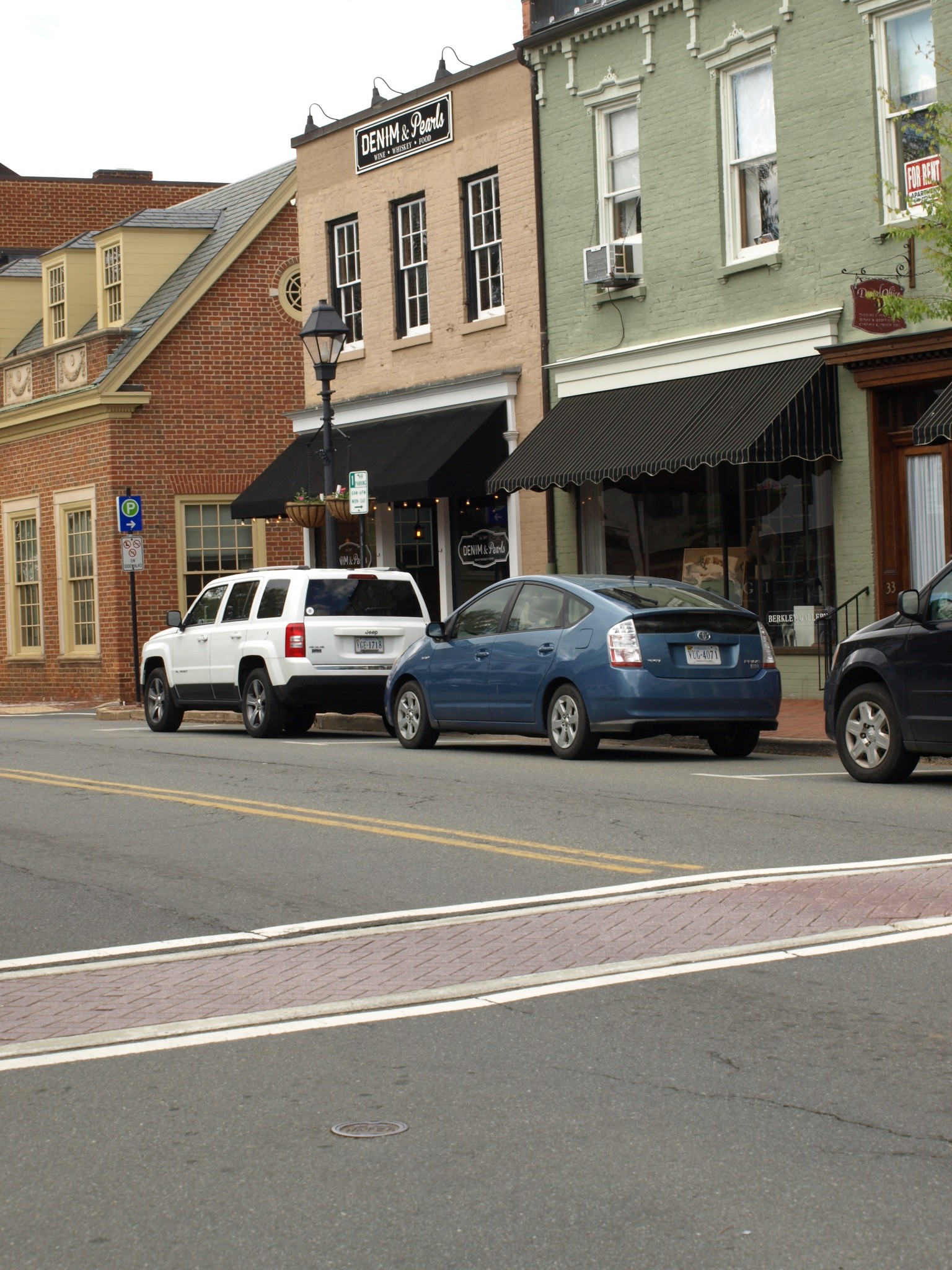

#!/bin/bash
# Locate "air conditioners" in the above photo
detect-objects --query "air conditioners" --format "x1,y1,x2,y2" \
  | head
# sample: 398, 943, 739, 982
583, 241, 635, 283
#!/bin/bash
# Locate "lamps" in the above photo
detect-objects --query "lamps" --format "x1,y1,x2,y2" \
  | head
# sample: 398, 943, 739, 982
412, 504, 426, 541
434, 46, 470, 81
369, 75, 405, 109
304, 103, 342, 134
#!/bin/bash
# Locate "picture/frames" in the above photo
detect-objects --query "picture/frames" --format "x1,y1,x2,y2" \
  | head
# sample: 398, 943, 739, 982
679, 544, 747, 607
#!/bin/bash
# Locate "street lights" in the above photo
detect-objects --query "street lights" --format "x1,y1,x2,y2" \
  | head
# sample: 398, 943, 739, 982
302, 298, 352, 568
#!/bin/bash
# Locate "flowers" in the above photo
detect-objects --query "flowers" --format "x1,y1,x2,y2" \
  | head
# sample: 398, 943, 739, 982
327, 486, 374, 500
290, 486, 326, 506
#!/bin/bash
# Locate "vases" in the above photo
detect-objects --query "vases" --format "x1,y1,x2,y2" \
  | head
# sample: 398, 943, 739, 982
327, 496, 378, 525
285, 502, 331, 529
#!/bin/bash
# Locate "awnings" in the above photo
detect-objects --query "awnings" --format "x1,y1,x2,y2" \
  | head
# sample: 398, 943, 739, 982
909, 381, 951, 451
483, 352, 845, 494
226, 399, 506, 518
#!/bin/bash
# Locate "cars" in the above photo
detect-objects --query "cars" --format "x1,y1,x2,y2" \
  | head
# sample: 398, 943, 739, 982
822, 556, 952, 784
386, 572, 781, 761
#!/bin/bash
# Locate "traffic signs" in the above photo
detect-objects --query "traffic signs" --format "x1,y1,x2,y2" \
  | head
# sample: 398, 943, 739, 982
349, 470, 370, 515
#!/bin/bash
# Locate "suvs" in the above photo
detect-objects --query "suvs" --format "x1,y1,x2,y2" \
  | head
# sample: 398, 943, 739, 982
139, 564, 433, 738
